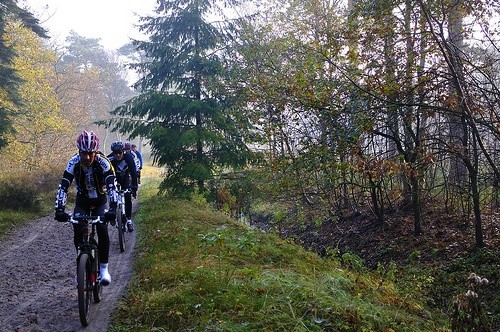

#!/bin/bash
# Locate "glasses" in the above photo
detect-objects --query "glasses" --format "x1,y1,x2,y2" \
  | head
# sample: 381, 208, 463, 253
113, 151, 122, 154
80, 150, 94, 155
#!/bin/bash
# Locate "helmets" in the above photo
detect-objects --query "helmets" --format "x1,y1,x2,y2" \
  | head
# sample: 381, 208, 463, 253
131, 144, 137, 148
111, 141, 124, 151
124, 143, 132, 149
76, 130, 100, 152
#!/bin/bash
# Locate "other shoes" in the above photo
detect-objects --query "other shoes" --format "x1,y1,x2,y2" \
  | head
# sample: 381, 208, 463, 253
110, 219, 116, 226
127, 222, 133, 232
75, 272, 86, 287
99, 268, 111, 286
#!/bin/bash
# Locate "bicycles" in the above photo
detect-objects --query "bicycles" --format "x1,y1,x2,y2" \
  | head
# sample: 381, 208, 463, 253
108, 183, 135, 253
54, 205, 115, 327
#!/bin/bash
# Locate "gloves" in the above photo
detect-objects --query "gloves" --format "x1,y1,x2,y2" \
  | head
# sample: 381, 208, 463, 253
55, 208, 68, 222
131, 185, 137, 192
104, 204, 117, 221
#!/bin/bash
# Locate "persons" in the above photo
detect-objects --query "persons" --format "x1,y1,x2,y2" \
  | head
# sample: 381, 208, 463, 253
124, 142, 143, 196
54, 130, 117, 290
104, 141, 137, 232
